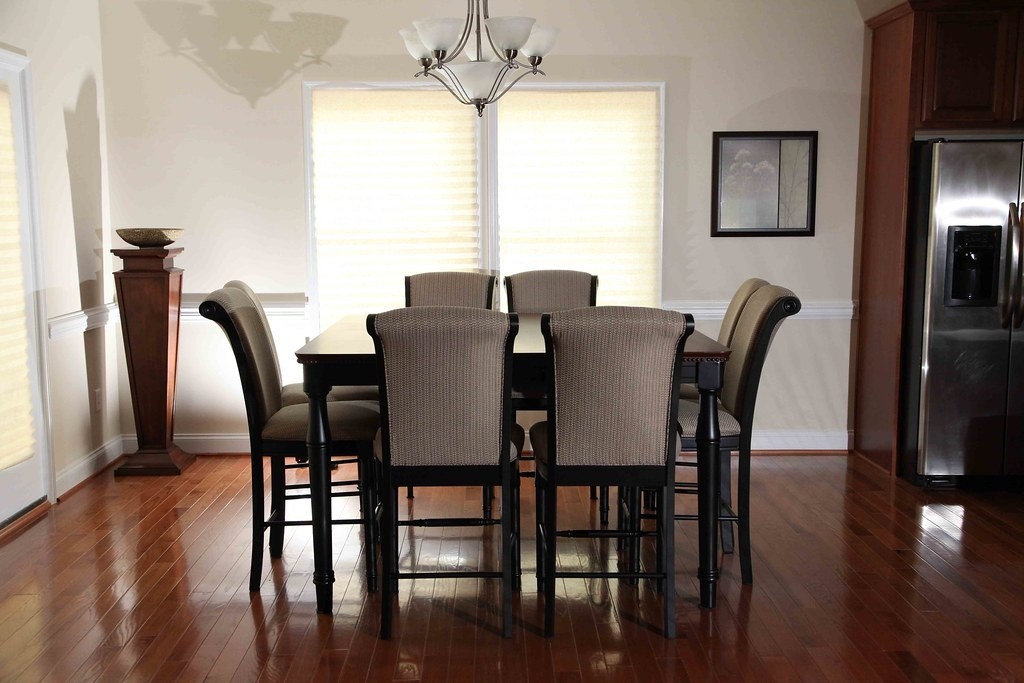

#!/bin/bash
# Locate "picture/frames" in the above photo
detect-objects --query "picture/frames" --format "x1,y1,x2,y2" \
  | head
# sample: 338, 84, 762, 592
711, 130, 816, 240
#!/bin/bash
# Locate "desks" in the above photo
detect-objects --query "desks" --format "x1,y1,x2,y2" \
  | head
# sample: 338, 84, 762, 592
292, 315, 735, 612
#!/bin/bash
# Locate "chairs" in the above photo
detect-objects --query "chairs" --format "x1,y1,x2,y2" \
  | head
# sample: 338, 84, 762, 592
402, 272, 498, 515
616, 277, 772, 554
503, 270, 613, 530
530, 305, 697, 640
624, 284, 802, 584
199, 280, 388, 595
365, 303, 522, 642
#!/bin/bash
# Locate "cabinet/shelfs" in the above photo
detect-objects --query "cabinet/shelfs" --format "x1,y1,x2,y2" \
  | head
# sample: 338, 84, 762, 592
853, 0, 1024, 507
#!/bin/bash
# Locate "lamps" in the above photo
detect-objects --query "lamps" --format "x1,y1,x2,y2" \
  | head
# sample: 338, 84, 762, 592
400, 0, 561, 121
